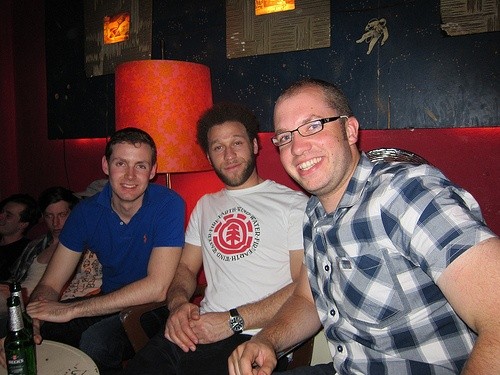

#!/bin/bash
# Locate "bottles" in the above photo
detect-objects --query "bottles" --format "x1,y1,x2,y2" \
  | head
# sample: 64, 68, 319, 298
9, 281, 35, 351
4, 296, 37, 375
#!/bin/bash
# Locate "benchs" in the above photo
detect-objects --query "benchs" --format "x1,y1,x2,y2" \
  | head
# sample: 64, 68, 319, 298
25, 128, 500, 368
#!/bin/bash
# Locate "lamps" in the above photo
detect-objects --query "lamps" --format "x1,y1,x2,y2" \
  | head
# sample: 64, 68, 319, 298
115, 60, 215, 189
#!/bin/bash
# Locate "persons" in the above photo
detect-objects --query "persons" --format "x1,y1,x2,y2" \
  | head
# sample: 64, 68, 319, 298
0, 189, 103, 338
227, 80, 500, 375
356, 18, 389, 55
0, 193, 42, 283
0, 127, 186, 375
124, 104, 309, 375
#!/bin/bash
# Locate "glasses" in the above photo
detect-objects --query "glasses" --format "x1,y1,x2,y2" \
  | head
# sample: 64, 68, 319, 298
271, 116, 349, 147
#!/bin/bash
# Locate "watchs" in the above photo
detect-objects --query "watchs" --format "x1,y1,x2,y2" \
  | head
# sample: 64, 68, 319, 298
229, 308, 245, 334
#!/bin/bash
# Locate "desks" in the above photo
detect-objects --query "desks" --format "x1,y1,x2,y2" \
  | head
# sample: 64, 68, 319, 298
0, 340, 100, 375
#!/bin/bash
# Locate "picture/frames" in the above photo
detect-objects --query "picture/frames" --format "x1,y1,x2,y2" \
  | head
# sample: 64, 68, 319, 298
226, 0, 331, 58
441, 0, 500, 34
84, 0, 152, 76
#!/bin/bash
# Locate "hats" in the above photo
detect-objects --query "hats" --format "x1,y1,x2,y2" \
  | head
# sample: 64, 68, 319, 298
73, 180, 107, 196
